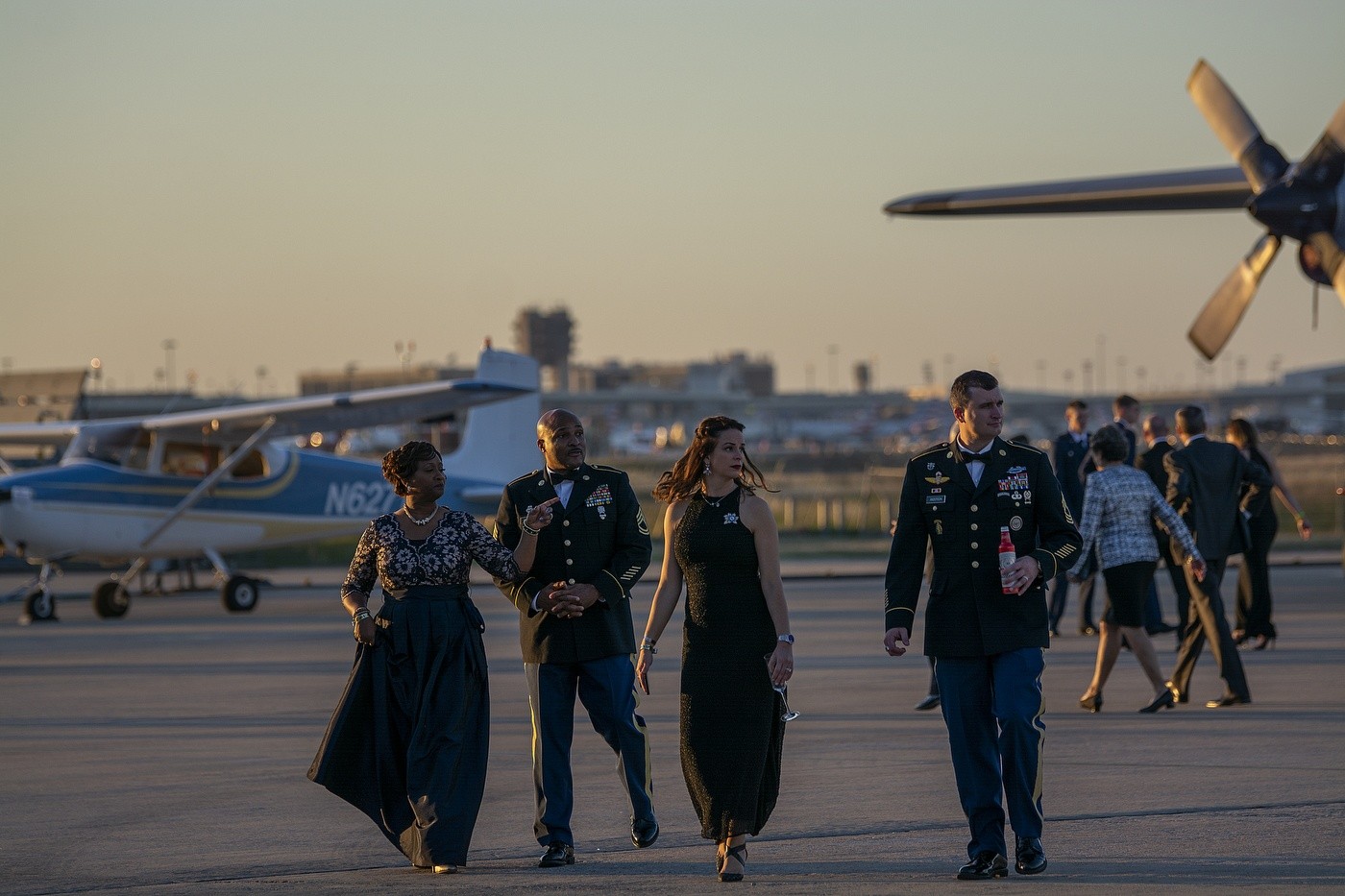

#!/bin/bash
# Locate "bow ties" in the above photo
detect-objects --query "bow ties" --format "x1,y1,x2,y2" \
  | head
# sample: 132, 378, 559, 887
957, 451, 993, 465
550, 469, 579, 485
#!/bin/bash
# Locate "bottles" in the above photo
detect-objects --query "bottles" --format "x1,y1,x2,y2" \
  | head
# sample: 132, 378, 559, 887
998, 525, 1019, 595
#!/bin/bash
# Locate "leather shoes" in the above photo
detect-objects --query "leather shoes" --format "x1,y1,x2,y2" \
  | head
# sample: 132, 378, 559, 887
1206, 692, 1250, 708
1015, 835, 1048, 875
538, 842, 575, 868
958, 850, 1008, 880
630, 814, 660, 849
1164, 679, 1188, 702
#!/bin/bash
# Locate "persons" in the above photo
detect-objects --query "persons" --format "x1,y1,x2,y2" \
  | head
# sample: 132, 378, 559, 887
306, 439, 560, 874
889, 396, 1311, 714
491, 409, 659, 868
884, 370, 1084, 880
634, 415, 795, 883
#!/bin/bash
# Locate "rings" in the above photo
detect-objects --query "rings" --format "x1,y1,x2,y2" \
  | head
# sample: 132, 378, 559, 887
546, 507, 552, 513
885, 646, 889, 651
526, 506, 533, 513
786, 668, 792, 672
1023, 576, 1028, 581
550, 513, 553, 520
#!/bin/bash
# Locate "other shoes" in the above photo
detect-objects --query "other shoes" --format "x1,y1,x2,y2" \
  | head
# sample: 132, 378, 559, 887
915, 695, 940, 711
1050, 629, 1059, 638
1079, 625, 1099, 636
432, 864, 457, 874
1145, 623, 1178, 635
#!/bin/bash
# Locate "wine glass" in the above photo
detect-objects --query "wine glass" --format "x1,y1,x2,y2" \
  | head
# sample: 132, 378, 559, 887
764, 654, 801, 722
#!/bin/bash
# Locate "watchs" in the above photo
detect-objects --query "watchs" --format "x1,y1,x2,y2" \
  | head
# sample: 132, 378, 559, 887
779, 634, 795, 644
1036, 561, 1042, 572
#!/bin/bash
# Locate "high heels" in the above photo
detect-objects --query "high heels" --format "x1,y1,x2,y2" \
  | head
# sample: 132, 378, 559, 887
1246, 633, 1276, 650
715, 838, 727, 873
1231, 629, 1250, 649
1080, 692, 1102, 711
718, 843, 748, 882
1140, 688, 1174, 713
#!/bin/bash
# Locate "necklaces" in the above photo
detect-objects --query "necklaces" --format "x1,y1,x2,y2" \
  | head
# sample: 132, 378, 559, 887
403, 502, 438, 526
700, 485, 738, 508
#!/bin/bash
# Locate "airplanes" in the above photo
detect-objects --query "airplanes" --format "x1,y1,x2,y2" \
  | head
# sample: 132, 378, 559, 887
1, 345, 546, 628
880, 58, 1345, 365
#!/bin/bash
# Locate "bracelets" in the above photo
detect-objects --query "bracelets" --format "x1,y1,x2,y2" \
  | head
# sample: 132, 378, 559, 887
351, 606, 370, 627
640, 645, 657, 654
644, 637, 656, 645
522, 519, 540, 536
